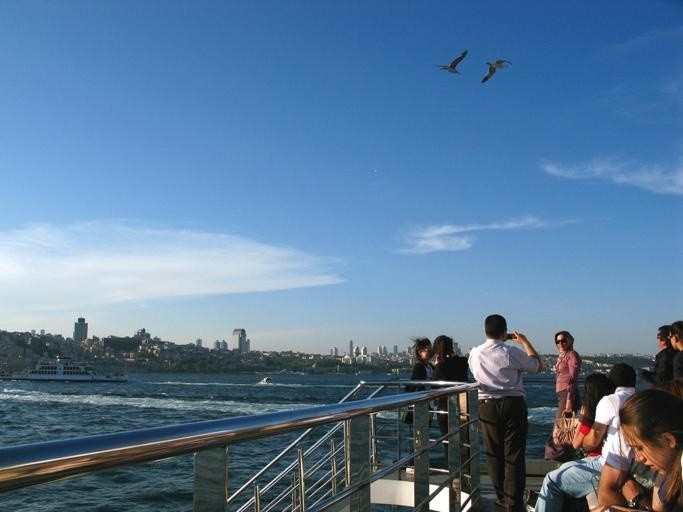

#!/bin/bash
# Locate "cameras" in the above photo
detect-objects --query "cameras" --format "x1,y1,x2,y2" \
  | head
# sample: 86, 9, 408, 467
507, 333, 516, 340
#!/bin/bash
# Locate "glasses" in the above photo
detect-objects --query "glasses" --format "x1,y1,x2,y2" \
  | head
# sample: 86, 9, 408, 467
556, 339, 566, 344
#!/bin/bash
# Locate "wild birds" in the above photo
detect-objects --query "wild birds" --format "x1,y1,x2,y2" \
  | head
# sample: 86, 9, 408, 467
433, 49, 468, 75
480, 59, 512, 84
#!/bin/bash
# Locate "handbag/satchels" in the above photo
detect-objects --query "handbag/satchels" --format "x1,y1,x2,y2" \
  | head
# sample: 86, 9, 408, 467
401, 405, 433, 426
551, 409, 581, 449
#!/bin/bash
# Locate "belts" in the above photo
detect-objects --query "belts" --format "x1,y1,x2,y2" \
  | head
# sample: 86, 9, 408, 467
478, 395, 523, 404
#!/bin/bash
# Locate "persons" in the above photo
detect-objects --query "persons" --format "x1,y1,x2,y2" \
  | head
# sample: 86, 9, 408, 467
467, 314, 544, 512
531, 321, 683, 512
429, 335, 468, 475
400, 338, 435, 425
